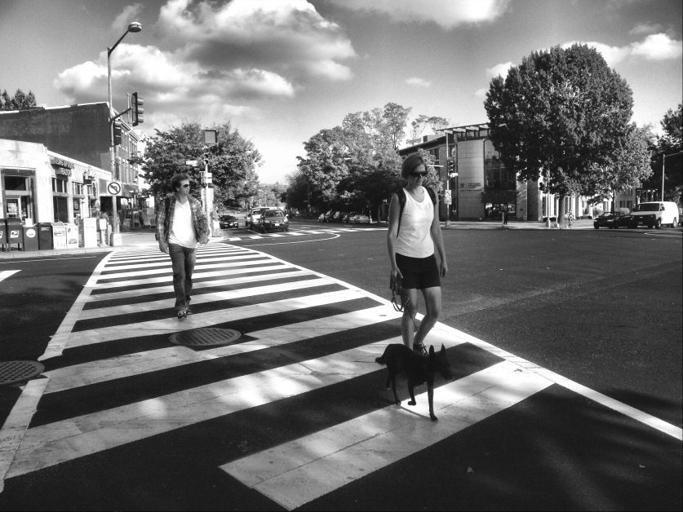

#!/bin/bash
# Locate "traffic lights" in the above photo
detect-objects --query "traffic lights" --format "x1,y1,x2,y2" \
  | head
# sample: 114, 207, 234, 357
203, 129, 218, 144
130, 91, 143, 126
447, 160, 453, 173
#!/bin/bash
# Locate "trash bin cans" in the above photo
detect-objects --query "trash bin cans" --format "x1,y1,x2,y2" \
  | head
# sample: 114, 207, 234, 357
0, 218, 52, 251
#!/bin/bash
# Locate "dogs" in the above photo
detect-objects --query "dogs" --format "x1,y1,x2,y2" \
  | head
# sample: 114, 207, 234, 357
374, 343, 454, 421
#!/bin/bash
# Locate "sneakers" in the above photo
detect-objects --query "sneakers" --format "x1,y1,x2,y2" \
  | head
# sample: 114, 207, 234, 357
177, 309, 186, 320
413, 342, 426, 356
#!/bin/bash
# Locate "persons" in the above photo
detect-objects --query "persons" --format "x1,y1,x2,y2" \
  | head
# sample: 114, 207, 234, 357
387, 154, 449, 351
153, 174, 209, 318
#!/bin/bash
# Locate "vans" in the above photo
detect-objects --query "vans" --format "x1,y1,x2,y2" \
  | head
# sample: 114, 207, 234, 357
627, 200, 680, 229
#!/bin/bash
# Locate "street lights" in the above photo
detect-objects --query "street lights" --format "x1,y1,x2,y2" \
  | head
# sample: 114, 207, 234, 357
105, 19, 142, 247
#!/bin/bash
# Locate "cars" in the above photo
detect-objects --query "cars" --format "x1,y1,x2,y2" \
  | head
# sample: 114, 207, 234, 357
219, 205, 292, 234
316, 210, 372, 225
594, 211, 629, 229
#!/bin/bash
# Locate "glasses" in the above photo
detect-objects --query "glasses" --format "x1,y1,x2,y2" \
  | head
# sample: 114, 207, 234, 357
410, 170, 428, 178
183, 184, 190, 187
